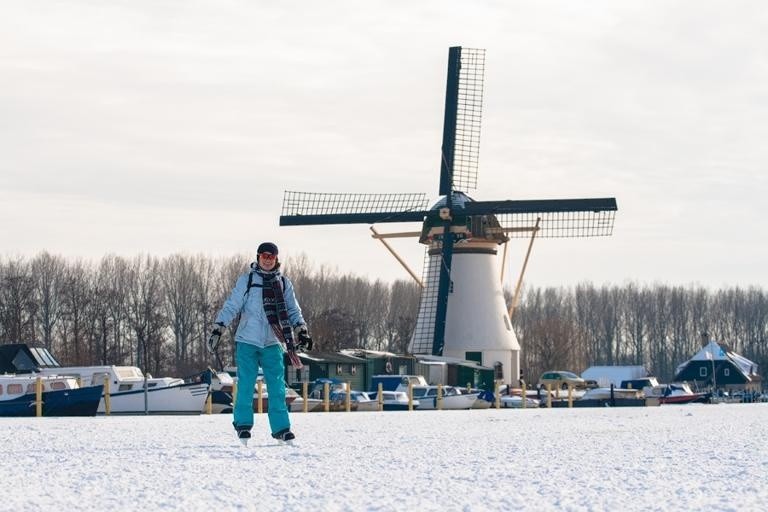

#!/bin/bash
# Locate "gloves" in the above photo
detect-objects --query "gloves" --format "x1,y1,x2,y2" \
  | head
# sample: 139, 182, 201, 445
206, 322, 226, 353
293, 322, 313, 353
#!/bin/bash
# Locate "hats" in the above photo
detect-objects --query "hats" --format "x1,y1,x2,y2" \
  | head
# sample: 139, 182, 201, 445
257, 243, 278, 261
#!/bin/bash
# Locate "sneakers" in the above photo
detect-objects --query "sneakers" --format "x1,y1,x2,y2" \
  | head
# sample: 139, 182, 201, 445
276, 432, 294, 441
237, 430, 251, 438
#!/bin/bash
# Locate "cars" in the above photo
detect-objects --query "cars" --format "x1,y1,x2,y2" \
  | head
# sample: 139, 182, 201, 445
539, 371, 588, 390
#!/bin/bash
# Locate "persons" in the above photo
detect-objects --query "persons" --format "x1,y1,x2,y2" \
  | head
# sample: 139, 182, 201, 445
207, 243, 313, 441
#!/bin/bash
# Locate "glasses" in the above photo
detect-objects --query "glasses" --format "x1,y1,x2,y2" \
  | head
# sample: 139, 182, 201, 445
257, 253, 277, 260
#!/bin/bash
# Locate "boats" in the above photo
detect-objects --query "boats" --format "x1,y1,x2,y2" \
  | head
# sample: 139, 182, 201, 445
0, 343, 212, 417
620, 378, 713, 403
